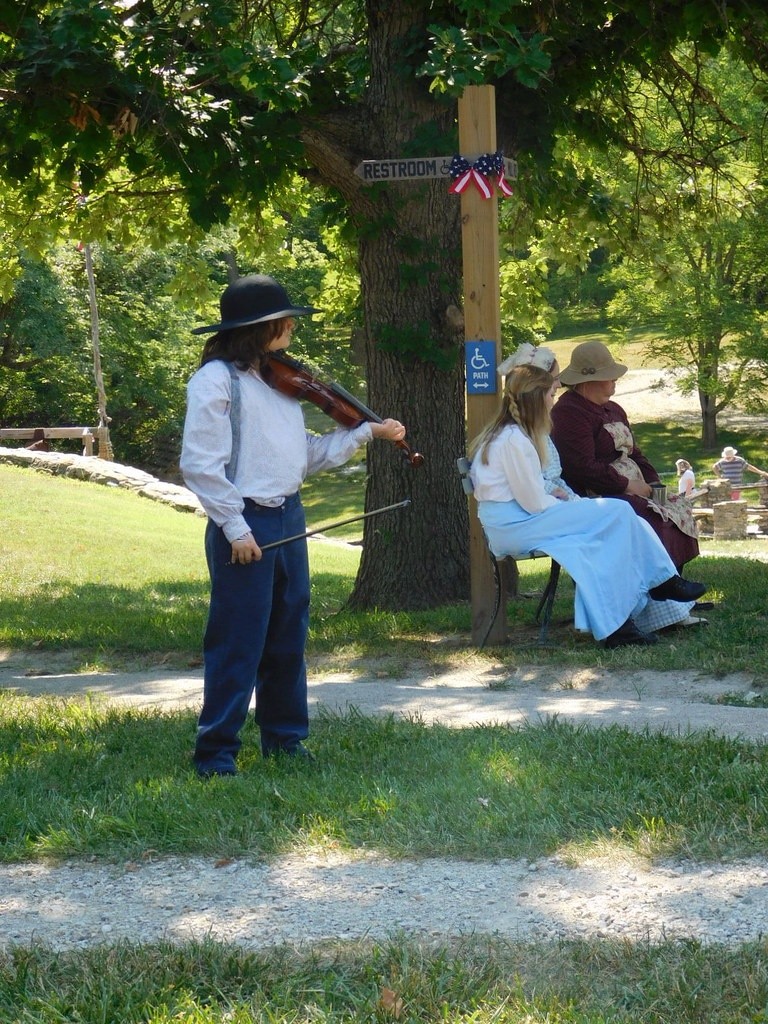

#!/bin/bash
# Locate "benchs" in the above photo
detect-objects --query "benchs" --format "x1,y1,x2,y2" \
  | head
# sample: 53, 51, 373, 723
455, 456, 561, 647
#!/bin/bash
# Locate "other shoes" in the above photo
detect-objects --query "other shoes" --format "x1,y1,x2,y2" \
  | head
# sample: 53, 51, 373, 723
677, 616, 708, 626
196, 762, 236, 779
691, 602, 714, 610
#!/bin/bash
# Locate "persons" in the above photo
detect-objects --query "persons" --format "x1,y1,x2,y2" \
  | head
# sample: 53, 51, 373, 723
549, 342, 714, 613
177, 275, 405, 787
469, 345, 706, 644
713, 447, 767, 501
675, 459, 697, 518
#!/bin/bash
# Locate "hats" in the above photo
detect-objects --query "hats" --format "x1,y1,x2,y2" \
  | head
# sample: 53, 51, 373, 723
190, 275, 324, 335
559, 342, 628, 385
722, 447, 737, 457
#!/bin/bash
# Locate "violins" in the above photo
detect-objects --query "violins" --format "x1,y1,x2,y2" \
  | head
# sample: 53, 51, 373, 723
260, 346, 425, 468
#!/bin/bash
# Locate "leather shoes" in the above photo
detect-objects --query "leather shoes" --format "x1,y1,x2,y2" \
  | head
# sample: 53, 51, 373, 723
649, 574, 706, 602
606, 615, 659, 649
